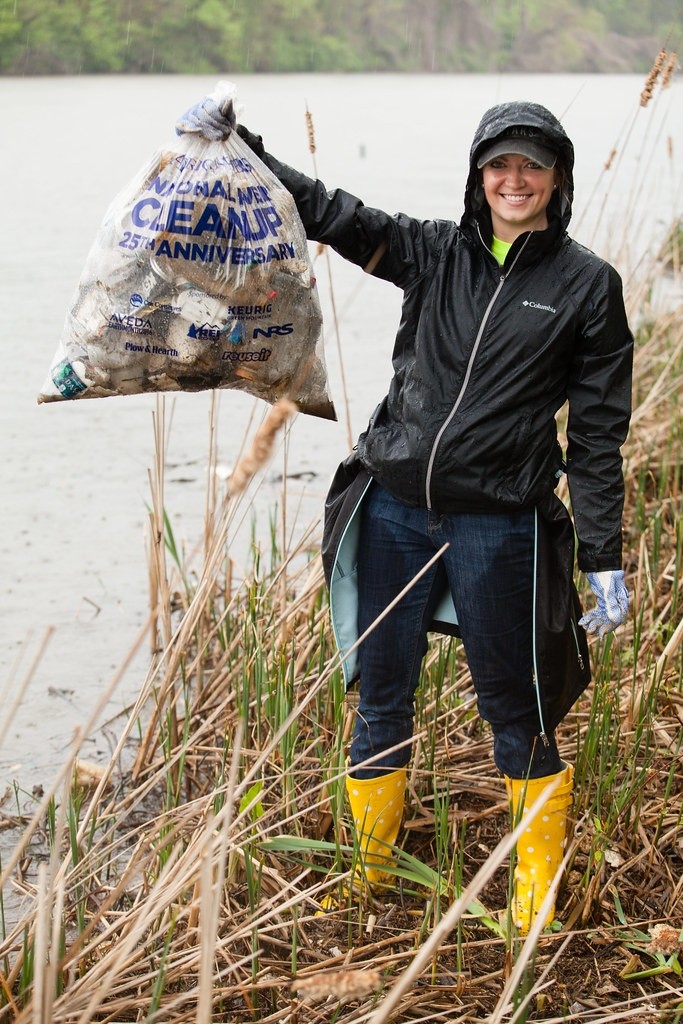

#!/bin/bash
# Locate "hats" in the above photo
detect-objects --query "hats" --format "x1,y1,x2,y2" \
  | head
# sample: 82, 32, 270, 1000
477, 126, 561, 170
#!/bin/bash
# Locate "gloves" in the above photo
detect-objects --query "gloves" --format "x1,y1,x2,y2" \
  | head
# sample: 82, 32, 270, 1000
577, 570, 629, 638
175, 95, 238, 142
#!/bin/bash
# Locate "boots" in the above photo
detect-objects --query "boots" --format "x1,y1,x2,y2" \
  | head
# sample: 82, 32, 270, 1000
314, 766, 407, 918
505, 759, 575, 935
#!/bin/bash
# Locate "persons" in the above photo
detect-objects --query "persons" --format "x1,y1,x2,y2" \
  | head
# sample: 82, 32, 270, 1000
175, 98, 634, 935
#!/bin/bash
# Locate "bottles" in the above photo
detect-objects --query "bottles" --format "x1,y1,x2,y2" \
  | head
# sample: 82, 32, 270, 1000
236, 273, 306, 381
170, 241, 269, 307
